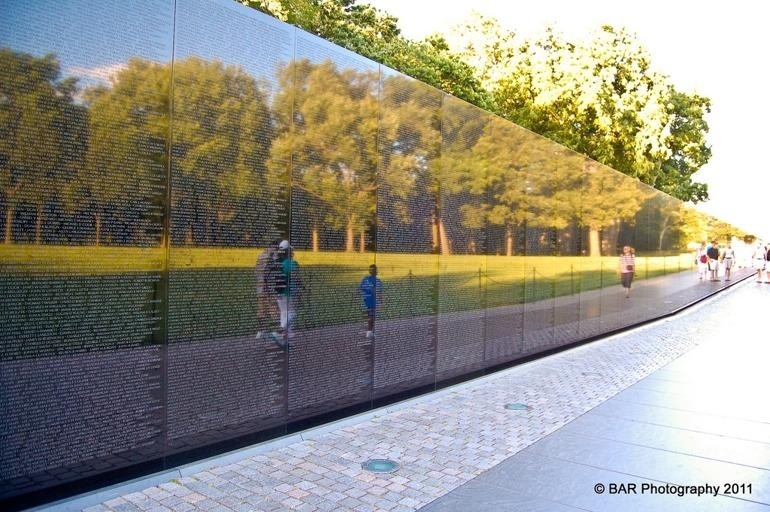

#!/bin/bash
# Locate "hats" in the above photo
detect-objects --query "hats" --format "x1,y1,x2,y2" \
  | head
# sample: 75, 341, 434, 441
278, 240, 294, 253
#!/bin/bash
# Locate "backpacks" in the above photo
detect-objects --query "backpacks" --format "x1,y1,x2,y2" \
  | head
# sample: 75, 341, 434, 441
264, 256, 286, 296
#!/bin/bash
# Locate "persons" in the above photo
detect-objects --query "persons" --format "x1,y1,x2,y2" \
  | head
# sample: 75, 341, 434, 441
271, 238, 301, 351
751, 242, 770, 284
360, 264, 383, 338
695, 236, 736, 281
252, 240, 280, 340
617, 245, 636, 295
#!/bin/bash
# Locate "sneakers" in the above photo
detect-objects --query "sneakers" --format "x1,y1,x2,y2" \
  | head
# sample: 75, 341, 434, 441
366, 330, 376, 339
698, 276, 732, 283
255, 329, 297, 341
